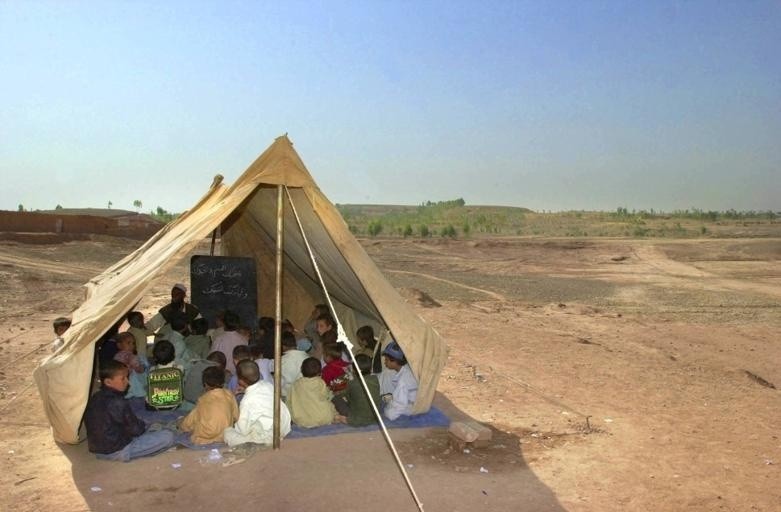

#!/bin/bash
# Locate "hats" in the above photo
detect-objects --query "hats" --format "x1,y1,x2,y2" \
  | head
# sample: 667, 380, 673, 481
380, 341, 404, 360
173, 284, 186, 293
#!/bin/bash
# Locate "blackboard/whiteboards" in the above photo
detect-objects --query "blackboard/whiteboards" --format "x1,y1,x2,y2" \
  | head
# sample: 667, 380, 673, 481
190, 255, 258, 330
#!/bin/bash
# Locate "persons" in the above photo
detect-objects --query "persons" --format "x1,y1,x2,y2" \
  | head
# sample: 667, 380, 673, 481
53, 316, 71, 351
